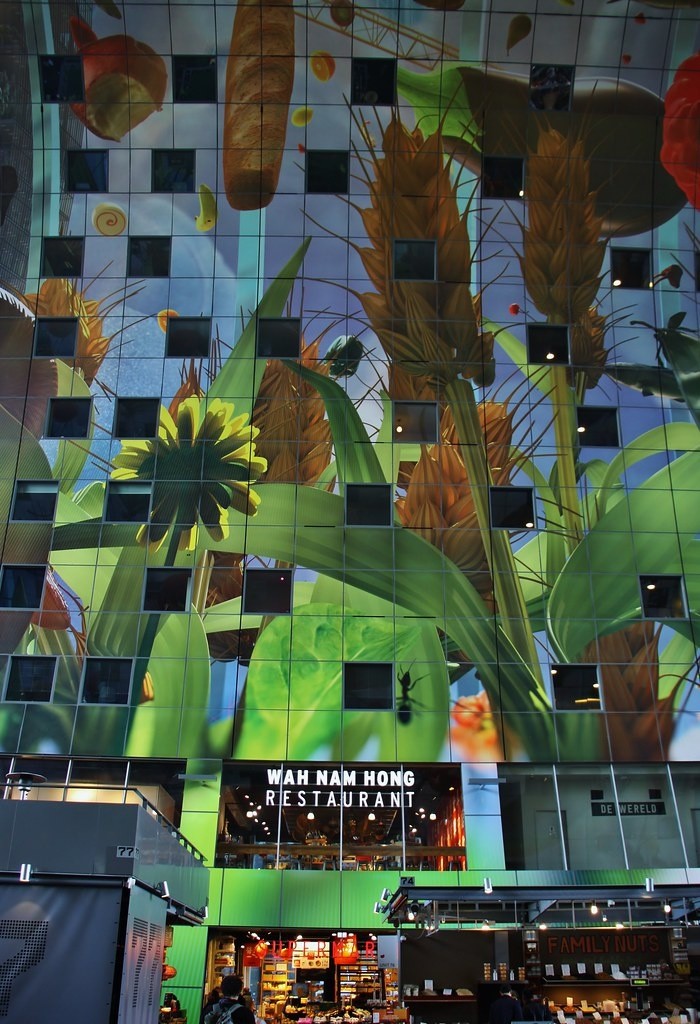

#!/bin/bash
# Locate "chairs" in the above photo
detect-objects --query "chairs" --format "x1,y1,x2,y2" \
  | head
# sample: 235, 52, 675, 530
252, 853, 390, 871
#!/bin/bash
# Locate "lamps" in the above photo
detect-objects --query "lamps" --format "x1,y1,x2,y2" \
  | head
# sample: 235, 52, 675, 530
234, 783, 271, 835
483, 919, 490, 931
307, 809, 316, 819
484, 877, 493, 893
20, 863, 32, 882
591, 901, 598, 915
442, 918, 447, 923
367, 810, 376, 820
374, 901, 384, 913
645, 877, 655, 893
664, 899, 672, 914
615, 920, 623, 929
380, 887, 394, 902
541, 922, 545, 929
198, 906, 209, 919
406, 908, 415, 921
240, 931, 378, 948
430, 811, 436, 820
603, 915, 606, 920
155, 881, 170, 898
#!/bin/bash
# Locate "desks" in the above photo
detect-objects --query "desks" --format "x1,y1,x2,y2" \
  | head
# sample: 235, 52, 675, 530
306, 861, 334, 870
216, 842, 467, 871
341, 859, 376, 871
6, 772, 47, 800
267, 858, 292, 870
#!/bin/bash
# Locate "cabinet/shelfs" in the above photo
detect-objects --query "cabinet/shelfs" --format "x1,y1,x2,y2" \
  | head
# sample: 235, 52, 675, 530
339, 956, 377, 1011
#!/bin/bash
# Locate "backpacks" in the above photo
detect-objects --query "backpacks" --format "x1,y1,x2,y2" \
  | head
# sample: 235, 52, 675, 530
204, 1003, 242, 1024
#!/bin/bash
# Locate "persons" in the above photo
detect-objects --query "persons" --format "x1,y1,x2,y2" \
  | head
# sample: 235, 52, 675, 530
200, 975, 267, 1024
488, 984, 553, 1024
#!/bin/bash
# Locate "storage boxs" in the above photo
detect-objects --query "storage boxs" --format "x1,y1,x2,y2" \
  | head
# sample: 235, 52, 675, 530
577, 1004, 598, 1015
549, 1004, 564, 1016
260, 950, 296, 1006
559, 1004, 581, 1016
